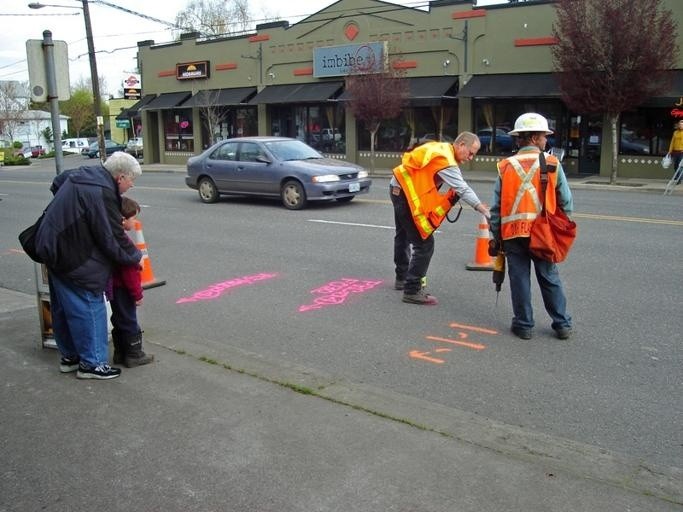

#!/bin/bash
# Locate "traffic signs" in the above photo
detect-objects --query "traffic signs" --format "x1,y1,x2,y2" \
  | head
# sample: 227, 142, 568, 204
113, 118, 130, 129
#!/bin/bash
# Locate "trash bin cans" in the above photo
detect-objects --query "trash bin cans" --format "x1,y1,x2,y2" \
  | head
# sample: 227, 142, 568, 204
566, 148, 579, 176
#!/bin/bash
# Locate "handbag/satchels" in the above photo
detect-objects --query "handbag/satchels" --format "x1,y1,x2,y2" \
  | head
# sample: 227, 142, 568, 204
17, 208, 51, 265
529, 205, 577, 264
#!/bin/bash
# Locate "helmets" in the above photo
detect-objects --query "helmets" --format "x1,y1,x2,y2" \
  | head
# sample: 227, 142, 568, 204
506, 112, 555, 137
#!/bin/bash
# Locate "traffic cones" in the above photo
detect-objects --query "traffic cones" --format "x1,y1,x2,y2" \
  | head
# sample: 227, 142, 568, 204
462, 212, 499, 272
129, 220, 167, 292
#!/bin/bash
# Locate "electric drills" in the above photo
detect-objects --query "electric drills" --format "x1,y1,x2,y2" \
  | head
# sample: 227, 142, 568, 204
487, 238, 506, 307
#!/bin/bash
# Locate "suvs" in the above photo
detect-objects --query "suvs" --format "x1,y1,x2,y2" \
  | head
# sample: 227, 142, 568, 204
124, 136, 143, 158
312, 126, 342, 141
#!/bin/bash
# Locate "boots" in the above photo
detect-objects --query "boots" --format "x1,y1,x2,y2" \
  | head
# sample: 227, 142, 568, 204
110, 330, 155, 369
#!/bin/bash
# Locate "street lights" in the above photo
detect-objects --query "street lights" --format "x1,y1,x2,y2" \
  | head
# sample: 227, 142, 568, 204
26, 1, 108, 166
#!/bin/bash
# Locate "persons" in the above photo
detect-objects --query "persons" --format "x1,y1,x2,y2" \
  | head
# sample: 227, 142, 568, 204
389, 131, 491, 305
668, 118, 683, 184
487, 112, 573, 339
106, 196, 154, 368
35, 151, 144, 379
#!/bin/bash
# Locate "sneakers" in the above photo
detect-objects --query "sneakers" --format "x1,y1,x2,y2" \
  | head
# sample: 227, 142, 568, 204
394, 279, 408, 290
552, 321, 573, 340
59, 358, 79, 373
509, 322, 535, 340
401, 291, 438, 305
76, 361, 121, 380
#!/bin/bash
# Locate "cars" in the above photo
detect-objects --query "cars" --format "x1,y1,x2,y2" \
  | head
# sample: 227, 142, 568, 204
12, 134, 126, 160
181, 135, 373, 210
407, 131, 456, 147
470, 124, 514, 150
544, 125, 649, 157
